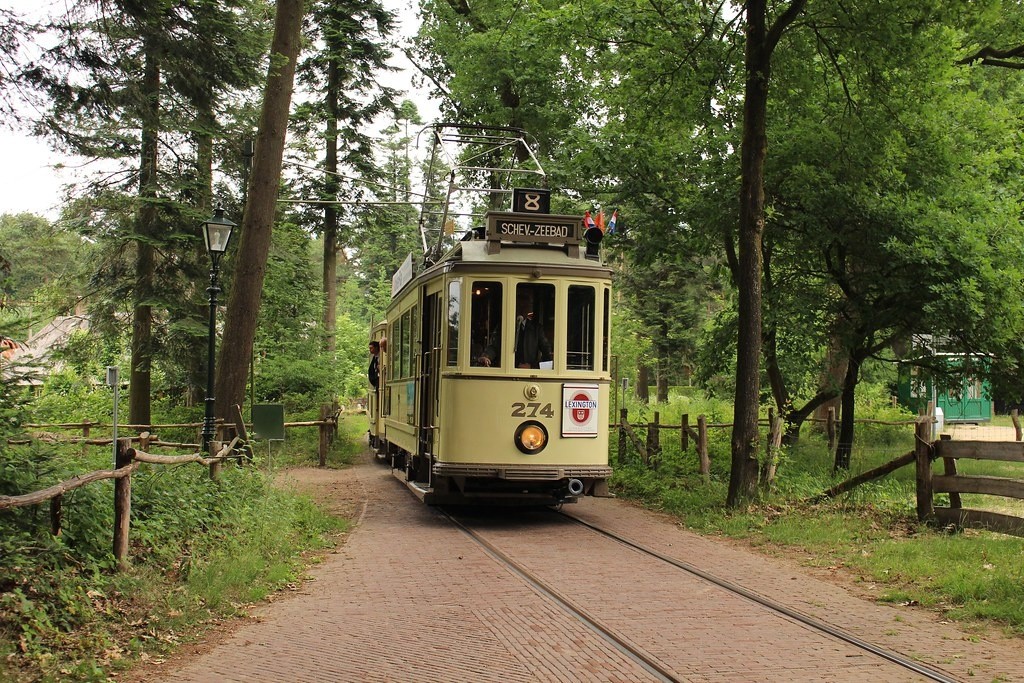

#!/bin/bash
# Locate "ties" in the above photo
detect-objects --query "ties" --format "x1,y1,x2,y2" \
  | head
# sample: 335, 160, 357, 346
519, 323, 524, 363
374, 356, 379, 373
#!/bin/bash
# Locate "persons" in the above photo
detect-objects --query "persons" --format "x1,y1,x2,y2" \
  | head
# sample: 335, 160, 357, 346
368, 340, 380, 403
379, 338, 387, 354
477, 290, 551, 369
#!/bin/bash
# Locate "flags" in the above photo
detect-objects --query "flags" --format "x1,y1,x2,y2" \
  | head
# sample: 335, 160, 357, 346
445, 220, 454, 237
584, 211, 596, 229
608, 210, 616, 236
594, 209, 604, 237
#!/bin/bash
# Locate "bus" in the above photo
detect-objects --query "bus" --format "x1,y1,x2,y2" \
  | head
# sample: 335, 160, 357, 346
364, 209, 615, 514
891, 352, 994, 426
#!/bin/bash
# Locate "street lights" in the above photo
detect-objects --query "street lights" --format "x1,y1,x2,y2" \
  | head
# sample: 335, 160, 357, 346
190, 202, 237, 456
